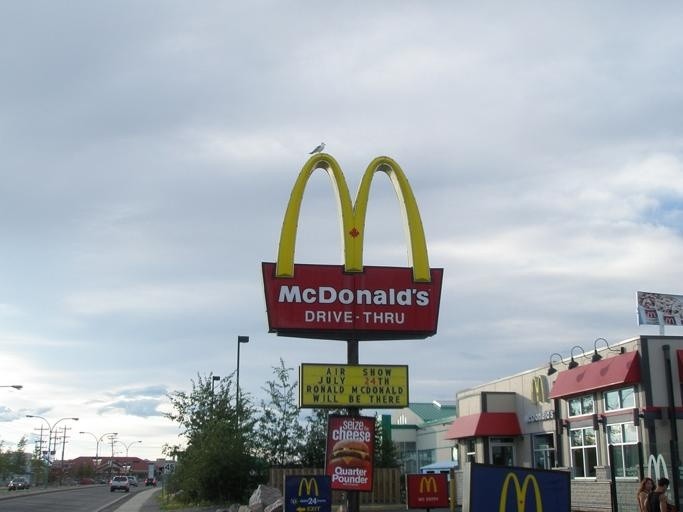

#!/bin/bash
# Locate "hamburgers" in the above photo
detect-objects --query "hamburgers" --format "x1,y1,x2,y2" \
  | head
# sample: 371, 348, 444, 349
329, 440, 370, 468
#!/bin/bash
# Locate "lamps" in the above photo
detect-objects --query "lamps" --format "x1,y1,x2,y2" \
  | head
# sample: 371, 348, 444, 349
557, 407, 649, 437
547, 337, 625, 376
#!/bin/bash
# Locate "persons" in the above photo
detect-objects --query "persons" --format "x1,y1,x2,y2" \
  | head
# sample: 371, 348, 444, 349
644, 477, 669, 511
637, 476, 656, 512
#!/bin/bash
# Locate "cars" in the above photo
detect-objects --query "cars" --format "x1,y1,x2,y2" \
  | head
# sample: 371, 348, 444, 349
144, 476, 157, 486
8, 477, 30, 490
128, 476, 138, 487
110, 476, 129, 492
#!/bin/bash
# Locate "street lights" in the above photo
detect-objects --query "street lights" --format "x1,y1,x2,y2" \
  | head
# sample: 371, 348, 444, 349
237, 335, 249, 413
26, 414, 79, 489
114, 440, 142, 475
79, 431, 117, 485
209, 375, 220, 419
